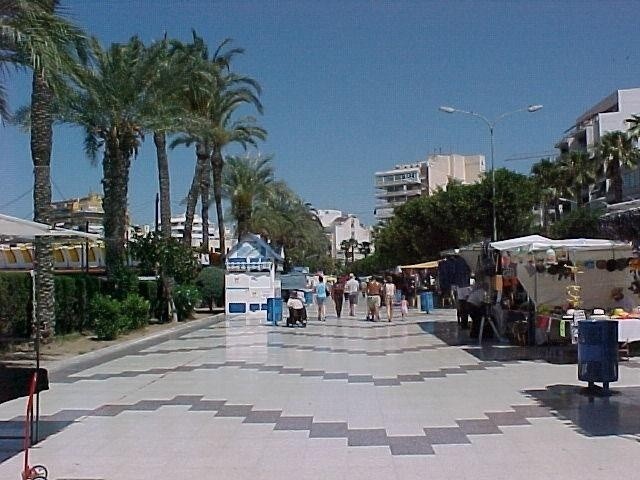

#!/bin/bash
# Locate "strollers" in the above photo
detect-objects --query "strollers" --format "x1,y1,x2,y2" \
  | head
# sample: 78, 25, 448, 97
365, 301, 374, 321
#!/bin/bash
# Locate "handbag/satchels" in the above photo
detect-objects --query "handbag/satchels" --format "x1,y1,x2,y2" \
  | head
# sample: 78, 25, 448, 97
325, 286, 330, 297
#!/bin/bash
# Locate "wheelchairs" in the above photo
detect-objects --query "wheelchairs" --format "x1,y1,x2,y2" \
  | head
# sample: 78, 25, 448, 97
287, 306, 307, 328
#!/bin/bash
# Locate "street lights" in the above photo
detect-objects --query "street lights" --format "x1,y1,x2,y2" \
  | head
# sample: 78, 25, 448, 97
440, 105, 544, 241
341, 239, 358, 262
360, 242, 372, 258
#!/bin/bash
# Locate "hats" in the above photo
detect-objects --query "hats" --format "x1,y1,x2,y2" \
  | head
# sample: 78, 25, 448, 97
537, 257, 639, 274
543, 249, 558, 265
590, 308, 640, 319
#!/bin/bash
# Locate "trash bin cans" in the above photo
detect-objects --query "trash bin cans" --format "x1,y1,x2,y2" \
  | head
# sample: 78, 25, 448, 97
574, 317, 620, 401
421, 292, 435, 316
266, 296, 283, 327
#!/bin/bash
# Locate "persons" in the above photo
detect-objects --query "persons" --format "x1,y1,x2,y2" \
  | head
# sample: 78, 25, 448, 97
466, 282, 489, 339
313, 273, 367, 321
457, 285, 472, 329
365, 275, 383, 322
382, 275, 397, 322
395, 295, 411, 319
